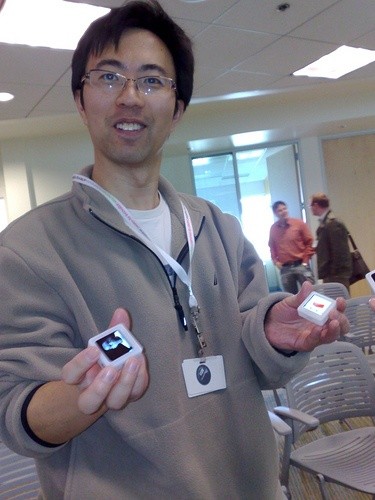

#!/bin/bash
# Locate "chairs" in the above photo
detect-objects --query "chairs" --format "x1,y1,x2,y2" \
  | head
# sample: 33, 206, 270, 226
0, 442, 41, 500
279, 341, 375, 500
344, 295, 375, 376
313, 282, 351, 300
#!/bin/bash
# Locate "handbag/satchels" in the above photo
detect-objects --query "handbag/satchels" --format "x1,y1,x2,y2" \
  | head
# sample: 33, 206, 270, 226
350, 251, 370, 285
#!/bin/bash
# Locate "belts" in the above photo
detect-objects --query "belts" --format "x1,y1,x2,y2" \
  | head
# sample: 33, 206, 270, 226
283, 260, 302, 267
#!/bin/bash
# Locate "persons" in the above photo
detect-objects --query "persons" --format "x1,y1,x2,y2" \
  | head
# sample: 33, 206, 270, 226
368, 297, 375, 312
309, 193, 352, 299
102, 331, 131, 350
268, 201, 315, 295
0, 0, 349, 500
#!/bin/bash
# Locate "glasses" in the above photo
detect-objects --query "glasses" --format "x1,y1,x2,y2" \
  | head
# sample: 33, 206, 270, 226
80, 67, 178, 93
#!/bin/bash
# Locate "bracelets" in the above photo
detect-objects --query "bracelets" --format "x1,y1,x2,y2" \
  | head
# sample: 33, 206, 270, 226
302, 262, 307, 265
